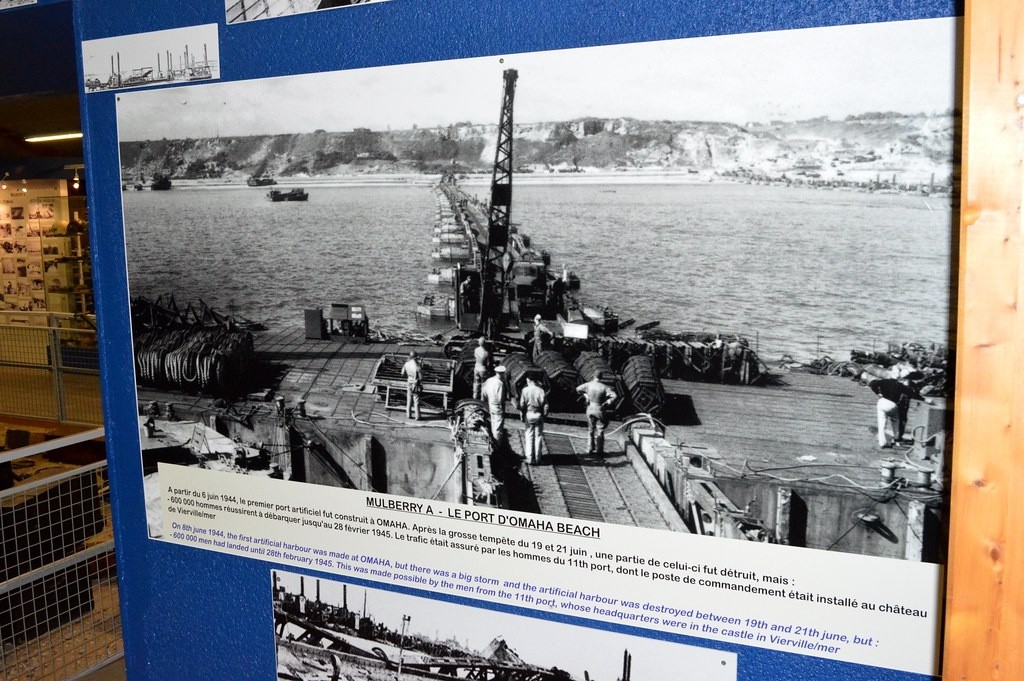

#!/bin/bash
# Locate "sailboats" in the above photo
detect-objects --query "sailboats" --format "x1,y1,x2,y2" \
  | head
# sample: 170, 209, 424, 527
106, 42, 213, 89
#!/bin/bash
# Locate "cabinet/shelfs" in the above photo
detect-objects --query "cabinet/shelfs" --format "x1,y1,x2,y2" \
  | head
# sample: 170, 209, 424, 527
37, 196, 100, 369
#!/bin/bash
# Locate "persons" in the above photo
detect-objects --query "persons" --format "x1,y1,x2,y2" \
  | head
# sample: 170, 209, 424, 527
400, 351, 426, 421
529, 313, 554, 364
481, 365, 508, 449
575, 369, 618, 457
519, 373, 549, 465
868, 366, 925, 449
473, 336, 490, 400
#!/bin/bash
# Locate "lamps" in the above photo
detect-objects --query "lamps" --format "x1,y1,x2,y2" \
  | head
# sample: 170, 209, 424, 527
0, 172, 9, 190
72, 168, 80, 189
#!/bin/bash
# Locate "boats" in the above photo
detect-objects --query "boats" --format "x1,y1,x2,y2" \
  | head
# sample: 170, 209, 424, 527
246, 177, 277, 187
150, 173, 172, 191
266, 186, 309, 203
328, 302, 368, 344
